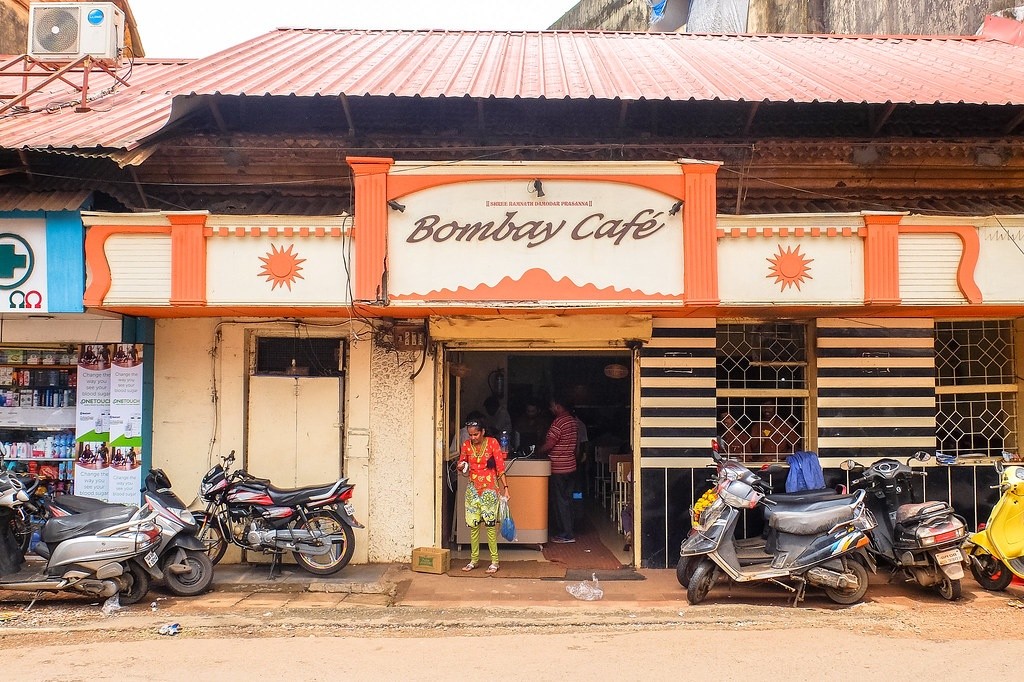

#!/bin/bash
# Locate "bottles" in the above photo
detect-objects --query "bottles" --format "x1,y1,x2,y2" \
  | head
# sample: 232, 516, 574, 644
0, 389, 74, 407
0, 434, 75, 497
500, 431, 509, 452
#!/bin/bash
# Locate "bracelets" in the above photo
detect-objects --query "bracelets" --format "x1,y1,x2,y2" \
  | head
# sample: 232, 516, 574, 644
504, 486, 508, 489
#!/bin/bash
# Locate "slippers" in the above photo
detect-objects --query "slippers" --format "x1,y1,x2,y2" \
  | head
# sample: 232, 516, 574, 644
462, 562, 479, 572
485, 564, 500, 574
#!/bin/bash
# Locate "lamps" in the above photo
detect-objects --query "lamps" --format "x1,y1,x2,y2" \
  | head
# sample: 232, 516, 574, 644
388, 201, 406, 213
604, 351, 628, 379
534, 180, 545, 197
668, 200, 683, 216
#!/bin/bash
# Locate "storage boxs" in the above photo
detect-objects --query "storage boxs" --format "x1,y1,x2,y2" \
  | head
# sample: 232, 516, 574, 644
0, 350, 77, 407
412, 547, 450, 575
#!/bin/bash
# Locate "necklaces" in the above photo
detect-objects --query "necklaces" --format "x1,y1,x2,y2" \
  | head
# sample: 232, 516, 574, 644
470, 440, 487, 463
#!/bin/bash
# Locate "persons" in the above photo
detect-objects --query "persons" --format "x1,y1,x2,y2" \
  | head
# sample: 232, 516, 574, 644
453, 418, 511, 576
113, 446, 139, 467
114, 345, 140, 366
539, 393, 588, 545
750, 400, 800, 462
514, 402, 547, 456
720, 412, 753, 462
79, 343, 111, 365
482, 395, 512, 439
78, 442, 109, 464
620, 470, 635, 551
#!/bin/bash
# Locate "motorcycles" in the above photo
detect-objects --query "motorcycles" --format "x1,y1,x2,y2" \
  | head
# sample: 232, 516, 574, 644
190, 448, 366, 582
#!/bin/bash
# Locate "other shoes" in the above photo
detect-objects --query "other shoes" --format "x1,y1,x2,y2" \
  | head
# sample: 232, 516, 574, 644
623, 534, 632, 551
551, 534, 576, 543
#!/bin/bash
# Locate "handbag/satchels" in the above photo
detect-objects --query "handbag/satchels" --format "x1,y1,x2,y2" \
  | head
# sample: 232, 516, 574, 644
501, 498, 516, 542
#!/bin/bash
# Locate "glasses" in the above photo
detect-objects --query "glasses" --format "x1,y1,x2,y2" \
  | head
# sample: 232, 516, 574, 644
465, 421, 484, 429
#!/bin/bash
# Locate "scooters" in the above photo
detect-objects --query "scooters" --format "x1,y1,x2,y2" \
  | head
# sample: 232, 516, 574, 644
0, 441, 213, 609
965, 457, 1024, 593
675, 435, 878, 607
839, 450, 971, 601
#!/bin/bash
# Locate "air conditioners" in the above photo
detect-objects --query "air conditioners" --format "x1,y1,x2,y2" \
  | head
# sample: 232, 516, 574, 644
27, 2, 126, 68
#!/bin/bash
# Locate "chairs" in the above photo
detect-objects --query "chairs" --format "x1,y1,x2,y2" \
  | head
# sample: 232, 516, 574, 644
594, 444, 632, 535
